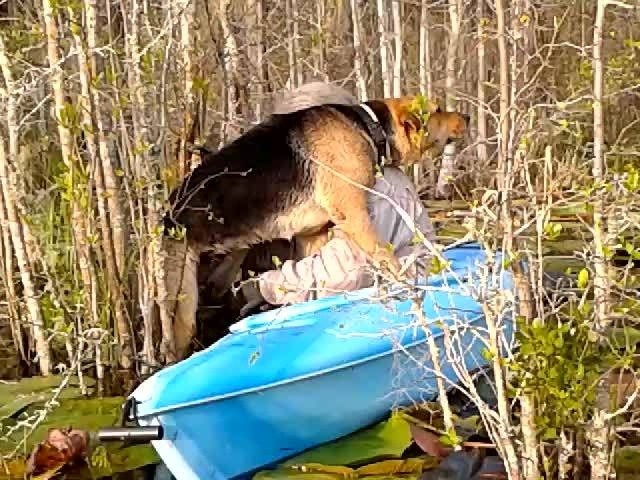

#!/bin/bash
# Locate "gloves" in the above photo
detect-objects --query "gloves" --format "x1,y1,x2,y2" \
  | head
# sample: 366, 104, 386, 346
236, 278, 266, 320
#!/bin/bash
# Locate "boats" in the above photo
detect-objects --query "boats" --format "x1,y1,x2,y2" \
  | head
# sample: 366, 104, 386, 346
119, 236, 528, 479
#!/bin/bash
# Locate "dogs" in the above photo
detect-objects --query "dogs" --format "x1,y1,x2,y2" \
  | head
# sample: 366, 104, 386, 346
150, 95, 470, 364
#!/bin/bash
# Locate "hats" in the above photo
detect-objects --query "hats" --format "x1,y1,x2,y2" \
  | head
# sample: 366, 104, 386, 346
273, 84, 361, 114
